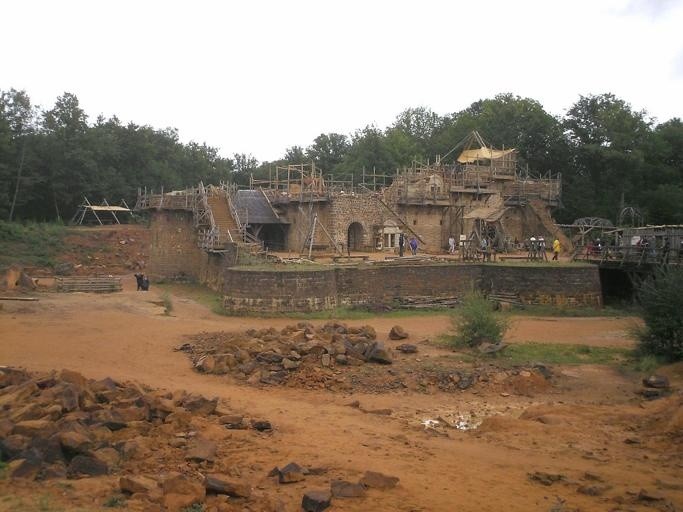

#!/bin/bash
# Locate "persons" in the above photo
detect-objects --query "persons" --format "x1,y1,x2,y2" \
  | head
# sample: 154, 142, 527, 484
636, 234, 683, 264
399, 232, 406, 257
552, 238, 560, 260
587, 237, 603, 256
448, 236, 455, 255
480, 236, 499, 262
529, 234, 548, 263
134, 272, 149, 291
409, 237, 418, 255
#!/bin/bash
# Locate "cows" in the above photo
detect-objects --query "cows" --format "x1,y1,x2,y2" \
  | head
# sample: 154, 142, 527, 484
134, 273, 149, 291
